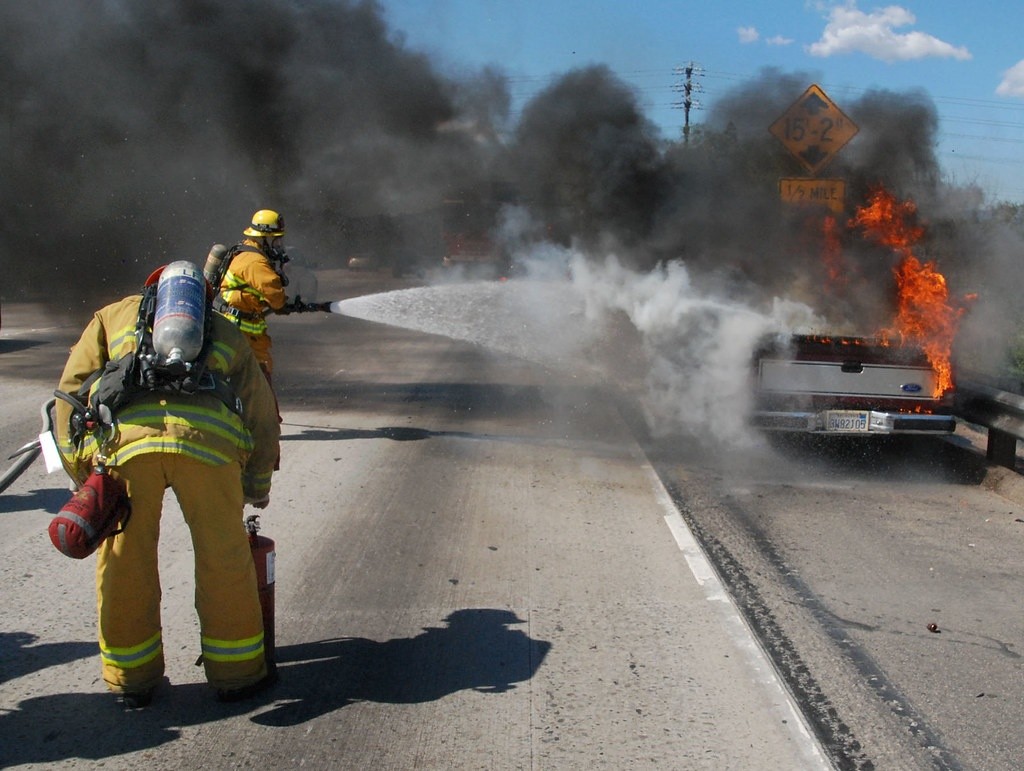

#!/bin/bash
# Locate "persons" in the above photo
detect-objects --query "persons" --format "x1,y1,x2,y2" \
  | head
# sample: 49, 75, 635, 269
211, 210, 291, 424
56, 261, 281, 711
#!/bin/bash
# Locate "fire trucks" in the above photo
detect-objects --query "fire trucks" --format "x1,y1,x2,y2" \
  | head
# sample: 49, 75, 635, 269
440, 211, 512, 278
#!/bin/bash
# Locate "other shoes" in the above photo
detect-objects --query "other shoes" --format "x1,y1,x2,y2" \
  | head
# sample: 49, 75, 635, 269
112, 675, 171, 709
218, 663, 277, 702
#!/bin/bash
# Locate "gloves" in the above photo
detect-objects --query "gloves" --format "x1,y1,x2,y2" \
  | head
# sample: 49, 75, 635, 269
242, 490, 270, 509
275, 304, 290, 316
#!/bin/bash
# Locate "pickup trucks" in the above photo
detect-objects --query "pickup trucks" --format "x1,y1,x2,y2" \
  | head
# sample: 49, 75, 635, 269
735, 332, 957, 441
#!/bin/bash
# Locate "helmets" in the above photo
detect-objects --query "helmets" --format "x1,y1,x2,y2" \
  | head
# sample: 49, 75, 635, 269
243, 210, 285, 237
145, 265, 214, 303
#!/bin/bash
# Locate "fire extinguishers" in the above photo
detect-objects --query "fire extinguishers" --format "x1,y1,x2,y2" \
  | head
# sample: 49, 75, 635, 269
245, 515, 276, 675
